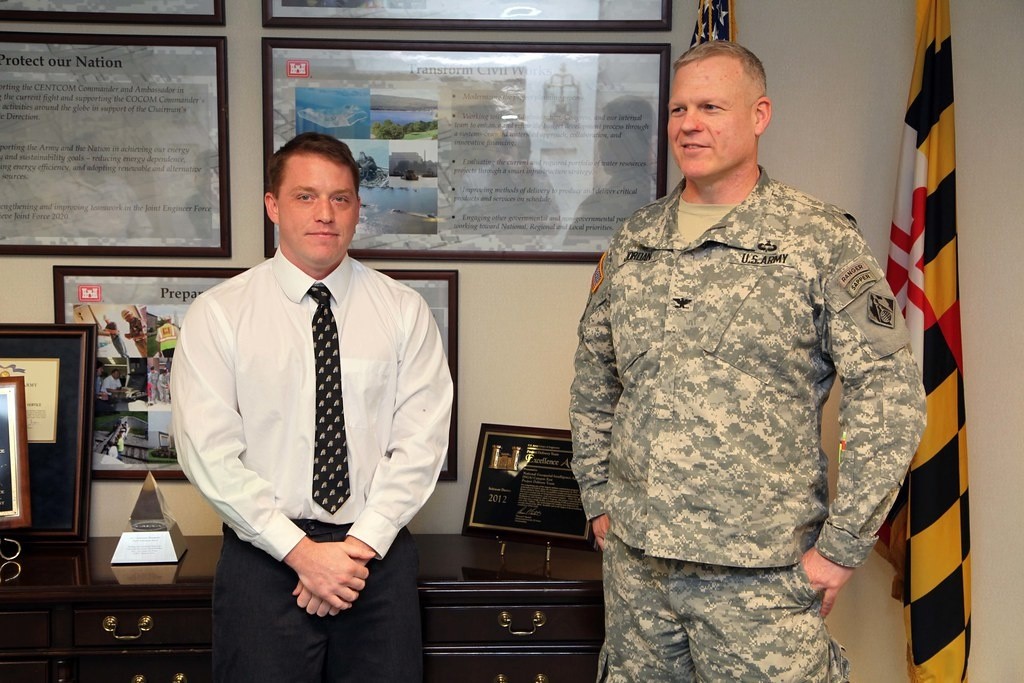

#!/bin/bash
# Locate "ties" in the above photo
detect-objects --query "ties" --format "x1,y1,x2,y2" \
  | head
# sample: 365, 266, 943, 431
307, 283, 351, 515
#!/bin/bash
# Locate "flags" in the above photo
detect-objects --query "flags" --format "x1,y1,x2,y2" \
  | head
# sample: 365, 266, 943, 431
840, 0, 972, 683
688, 0, 737, 48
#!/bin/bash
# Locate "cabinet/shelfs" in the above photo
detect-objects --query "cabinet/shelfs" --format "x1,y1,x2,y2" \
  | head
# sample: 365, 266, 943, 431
408, 534, 608, 683
0, 535, 223, 683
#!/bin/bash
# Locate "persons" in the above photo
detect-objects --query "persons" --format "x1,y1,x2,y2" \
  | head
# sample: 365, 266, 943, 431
569, 40, 928, 683
155, 316, 180, 358
147, 367, 171, 406
106, 419, 129, 461
451, 96, 653, 248
170, 132, 453, 683
97, 311, 146, 358
94, 363, 147, 412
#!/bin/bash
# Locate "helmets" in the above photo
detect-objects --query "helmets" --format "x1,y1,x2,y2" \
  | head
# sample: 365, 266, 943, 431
122, 310, 133, 319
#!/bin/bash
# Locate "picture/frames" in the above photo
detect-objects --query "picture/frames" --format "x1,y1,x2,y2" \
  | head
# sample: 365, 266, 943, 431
372, 268, 459, 482
0, 0, 226, 26
53, 265, 254, 481
260, 36, 671, 263
262, 0, 672, 31
0, 323, 98, 544
0, 31, 232, 258
460, 422, 599, 552
0, 375, 32, 530
